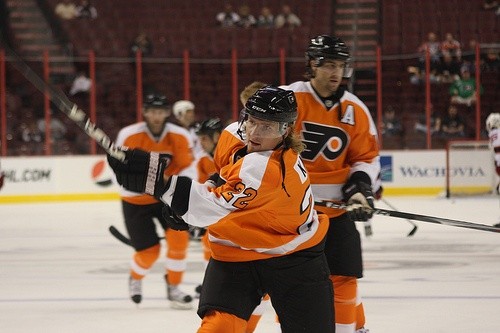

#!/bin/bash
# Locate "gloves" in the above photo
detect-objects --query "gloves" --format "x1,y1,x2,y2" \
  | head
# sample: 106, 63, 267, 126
106, 146, 173, 200
341, 179, 375, 222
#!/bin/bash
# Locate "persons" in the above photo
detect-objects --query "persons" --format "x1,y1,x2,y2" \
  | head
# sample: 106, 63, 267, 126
0, 0, 500, 333
115, 93, 194, 305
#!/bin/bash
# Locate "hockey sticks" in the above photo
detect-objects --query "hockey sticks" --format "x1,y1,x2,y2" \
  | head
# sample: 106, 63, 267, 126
378, 198, 417, 237
108, 225, 166, 247
0, 36, 124, 162
313, 197, 500, 233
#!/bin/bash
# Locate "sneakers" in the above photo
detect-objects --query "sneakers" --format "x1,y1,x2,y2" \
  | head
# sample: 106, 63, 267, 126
167, 285, 195, 310
128, 274, 143, 304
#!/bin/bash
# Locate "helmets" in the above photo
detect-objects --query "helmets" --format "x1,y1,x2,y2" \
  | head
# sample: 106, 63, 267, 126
486, 113, 499, 132
194, 114, 226, 136
247, 85, 298, 123
145, 92, 170, 108
173, 100, 194, 120
306, 33, 353, 63
